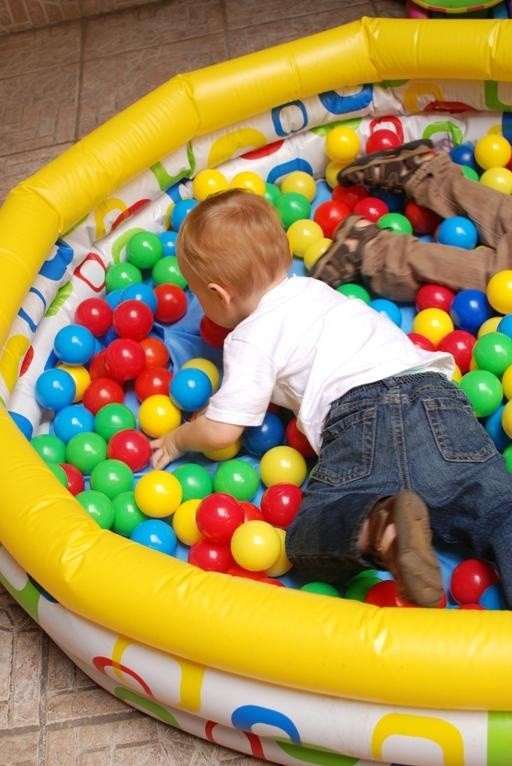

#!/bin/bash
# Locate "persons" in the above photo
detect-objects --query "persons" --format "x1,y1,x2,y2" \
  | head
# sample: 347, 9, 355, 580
148, 187, 512, 611
308, 139, 512, 302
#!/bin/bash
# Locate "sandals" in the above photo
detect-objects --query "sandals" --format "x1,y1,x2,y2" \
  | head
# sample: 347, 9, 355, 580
308, 216, 382, 288
337, 139, 436, 196
368, 492, 442, 606
